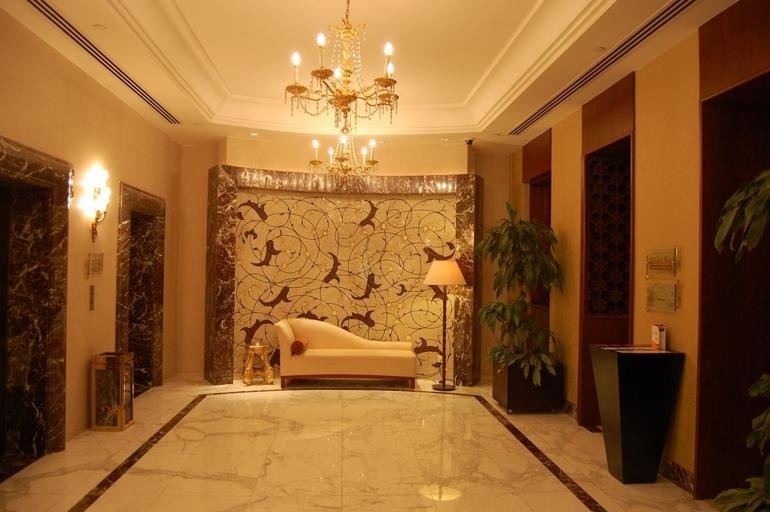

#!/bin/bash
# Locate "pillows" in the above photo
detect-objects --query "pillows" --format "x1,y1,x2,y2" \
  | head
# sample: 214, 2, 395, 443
291, 337, 308, 355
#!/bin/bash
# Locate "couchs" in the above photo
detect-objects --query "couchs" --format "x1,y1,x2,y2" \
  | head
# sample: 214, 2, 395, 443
273, 318, 417, 389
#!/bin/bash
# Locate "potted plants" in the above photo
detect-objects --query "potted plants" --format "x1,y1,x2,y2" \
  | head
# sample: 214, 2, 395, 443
475, 201, 563, 415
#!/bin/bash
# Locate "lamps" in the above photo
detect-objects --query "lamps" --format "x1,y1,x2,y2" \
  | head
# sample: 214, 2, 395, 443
78, 160, 111, 242
284, 0, 400, 192
423, 260, 467, 391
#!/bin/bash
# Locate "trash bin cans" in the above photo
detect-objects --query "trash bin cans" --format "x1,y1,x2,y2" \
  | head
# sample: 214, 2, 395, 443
91, 352, 134, 432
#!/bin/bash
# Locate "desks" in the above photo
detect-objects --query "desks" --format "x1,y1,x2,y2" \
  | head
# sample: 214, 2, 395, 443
591, 344, 686, 484
244, 345, 274, 385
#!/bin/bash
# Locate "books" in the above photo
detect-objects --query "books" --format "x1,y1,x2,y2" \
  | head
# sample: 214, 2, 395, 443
651, 324, 667, 351
